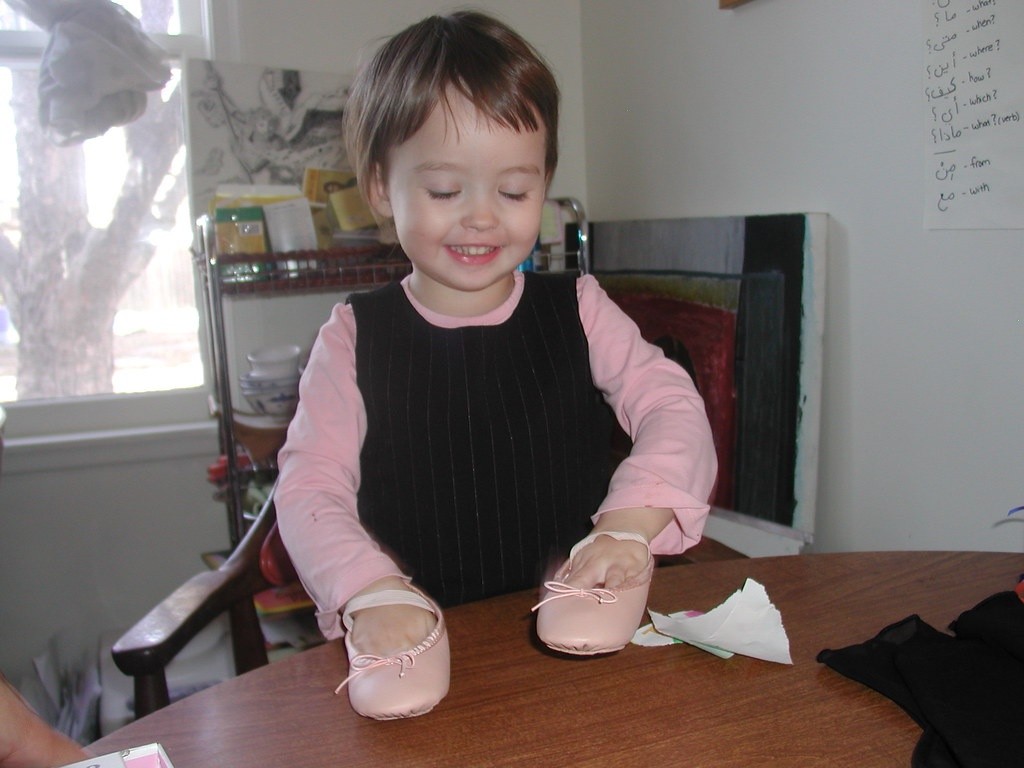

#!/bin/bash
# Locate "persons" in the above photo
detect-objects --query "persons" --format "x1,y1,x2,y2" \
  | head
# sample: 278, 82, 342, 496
275, 12, 719, 719
0, 674, 94, 768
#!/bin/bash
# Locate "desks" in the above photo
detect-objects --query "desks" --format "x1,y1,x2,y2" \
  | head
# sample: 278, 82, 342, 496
84, 550, 1024, 768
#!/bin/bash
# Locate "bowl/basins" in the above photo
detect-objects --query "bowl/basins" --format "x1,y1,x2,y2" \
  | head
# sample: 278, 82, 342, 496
240, 345, 302, 416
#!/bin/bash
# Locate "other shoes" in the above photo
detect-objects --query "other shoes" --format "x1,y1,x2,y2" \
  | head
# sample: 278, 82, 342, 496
335, 583, 451, 720
530, 532, 655, 656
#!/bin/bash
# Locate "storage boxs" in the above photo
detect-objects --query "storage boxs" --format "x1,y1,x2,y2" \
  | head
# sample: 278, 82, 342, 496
214, 206, 267, 284
325, 188, 385, 233
262, 197, 319, 279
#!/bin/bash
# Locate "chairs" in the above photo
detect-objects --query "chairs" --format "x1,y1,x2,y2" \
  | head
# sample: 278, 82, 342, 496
111, 473, 277, 721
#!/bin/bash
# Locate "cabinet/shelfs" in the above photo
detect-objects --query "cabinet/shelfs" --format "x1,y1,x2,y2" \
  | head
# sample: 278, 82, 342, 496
197, 197, 592, 573
